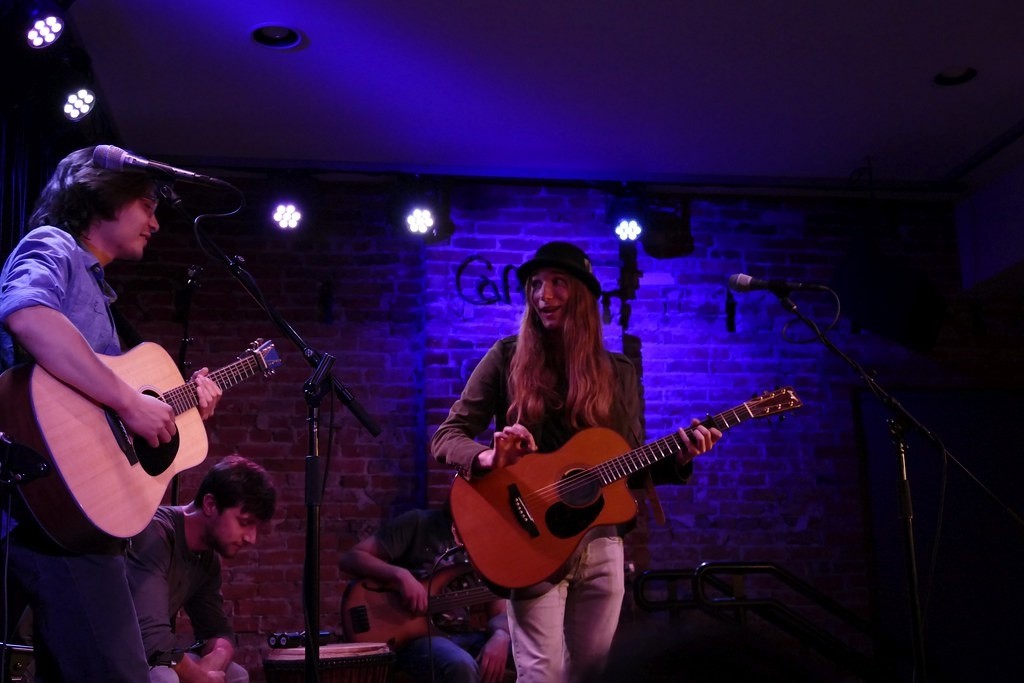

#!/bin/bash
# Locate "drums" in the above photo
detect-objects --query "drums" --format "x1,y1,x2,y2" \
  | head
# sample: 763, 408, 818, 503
261, 641, 389, 682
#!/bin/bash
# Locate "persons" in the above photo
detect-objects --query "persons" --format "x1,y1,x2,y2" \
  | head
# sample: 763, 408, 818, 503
429, 240, 722, 683
332, 510, 510, 683
123, 455, 281, 683
0, 145, 223, 683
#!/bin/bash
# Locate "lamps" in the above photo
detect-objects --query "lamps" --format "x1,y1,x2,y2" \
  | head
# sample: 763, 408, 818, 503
21, 1, 67, 51
611, 198, 647, 245
58, 74, 99, 124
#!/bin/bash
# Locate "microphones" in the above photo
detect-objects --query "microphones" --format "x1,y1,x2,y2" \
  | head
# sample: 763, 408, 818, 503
728, 272, 818, 292
93, 143, 210, 189
330, 375, 382, 440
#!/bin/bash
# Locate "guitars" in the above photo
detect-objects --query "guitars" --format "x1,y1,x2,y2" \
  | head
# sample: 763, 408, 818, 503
339, 563, 504, 651
448, 386, 807, 600
0, 337, 284, 555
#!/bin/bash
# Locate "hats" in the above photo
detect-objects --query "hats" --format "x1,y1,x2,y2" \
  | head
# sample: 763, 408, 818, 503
515, 240, 602, 301
